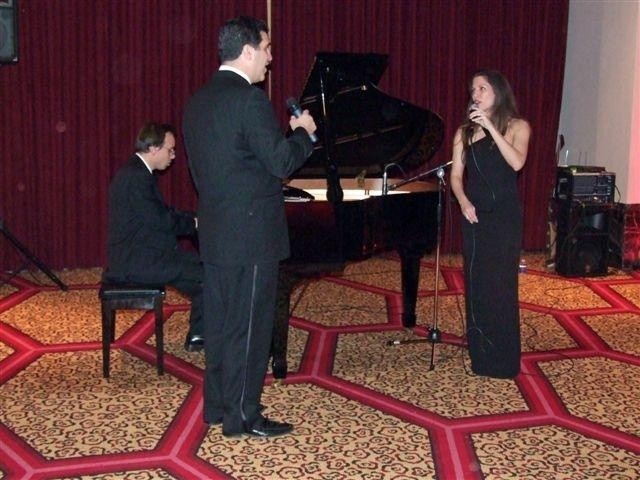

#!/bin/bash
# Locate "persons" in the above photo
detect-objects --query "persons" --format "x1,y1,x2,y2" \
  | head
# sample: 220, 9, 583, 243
451, 70, 531, 378
181, 14, 320, 439
106, 123, 208, 353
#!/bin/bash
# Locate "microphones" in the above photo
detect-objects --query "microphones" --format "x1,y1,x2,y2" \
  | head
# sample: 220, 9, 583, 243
466, 103, 479, 136
284, 97, 321, 147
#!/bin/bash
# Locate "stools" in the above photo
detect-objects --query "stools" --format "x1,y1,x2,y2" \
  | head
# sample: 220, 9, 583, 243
97, 282, 166, 377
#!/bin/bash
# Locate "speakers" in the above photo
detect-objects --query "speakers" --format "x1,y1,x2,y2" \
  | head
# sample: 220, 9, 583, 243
555, 200, 627, 277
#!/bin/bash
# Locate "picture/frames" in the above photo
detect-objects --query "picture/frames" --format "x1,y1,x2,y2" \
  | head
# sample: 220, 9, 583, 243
0, 0, 18, 64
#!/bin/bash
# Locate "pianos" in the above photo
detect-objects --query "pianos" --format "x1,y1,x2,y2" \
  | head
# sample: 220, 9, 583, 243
270, 51, 443, 379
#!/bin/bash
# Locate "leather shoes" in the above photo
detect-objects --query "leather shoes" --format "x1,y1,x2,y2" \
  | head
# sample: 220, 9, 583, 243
205, 404, 293, 436
185, 332, 206, 351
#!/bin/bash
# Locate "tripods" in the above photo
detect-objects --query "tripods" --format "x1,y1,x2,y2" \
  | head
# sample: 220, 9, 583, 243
386, 178, 469, 370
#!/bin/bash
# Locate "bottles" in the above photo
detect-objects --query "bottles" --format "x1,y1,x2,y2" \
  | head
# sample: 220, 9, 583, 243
518, 259, 527, 284
